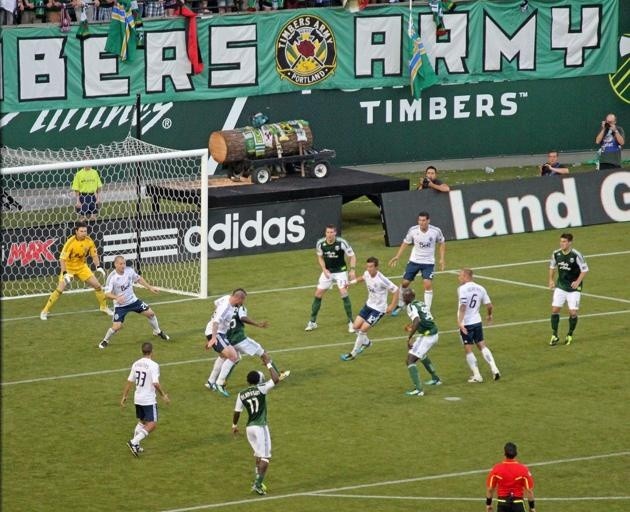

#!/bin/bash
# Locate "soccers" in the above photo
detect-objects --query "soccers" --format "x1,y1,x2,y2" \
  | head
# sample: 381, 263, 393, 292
256, 371, 266, 383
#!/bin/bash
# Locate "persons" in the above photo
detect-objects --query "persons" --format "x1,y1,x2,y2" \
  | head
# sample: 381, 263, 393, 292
414, 166, 451, 192
457, 269, 500, 385
549, 233, 590, 346
0, 0, 278, 26
71, 156, 102, 223
595, 114, 625, 171
485, 442, 536, 512
541, 149, 570, 176
120, 342, 170, 456
39, 220, 114, 316
303, 210, 445, 397
98, 257, 171, 349
232, 360, 281, 496
204, 288, 290, 397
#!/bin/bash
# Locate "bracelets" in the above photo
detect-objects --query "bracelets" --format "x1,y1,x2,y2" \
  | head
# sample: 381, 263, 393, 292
486, 498, 492, 505
97, 266, 102, 268
528, 500, 534, 510
613, 129, 618, 134
63, 271, 67, 275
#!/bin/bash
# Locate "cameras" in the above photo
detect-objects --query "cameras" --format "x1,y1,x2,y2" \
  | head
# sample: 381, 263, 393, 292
423, 177, 430, 188
542, 163, 551, 174
605, 123, 610, 128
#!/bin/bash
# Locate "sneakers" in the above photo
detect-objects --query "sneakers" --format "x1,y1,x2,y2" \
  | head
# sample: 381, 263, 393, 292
421, 378, 442, 387
466, 374, 484, 383
563, 334, 573, 346
250, 484, 267, 496
424, 312, 435, 321
205, 370, 291, 397
491, 368, 502, 379
40, 308, 170, 349
405, 389, 425, 397
126, 439, 145, 458
303, 319, 374, 362
550, 335, 559, 346
389, 305, 403, 317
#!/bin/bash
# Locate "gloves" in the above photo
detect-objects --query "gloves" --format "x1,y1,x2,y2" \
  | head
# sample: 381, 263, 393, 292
96, 267, 106, 277
62, 273, 74, 285
